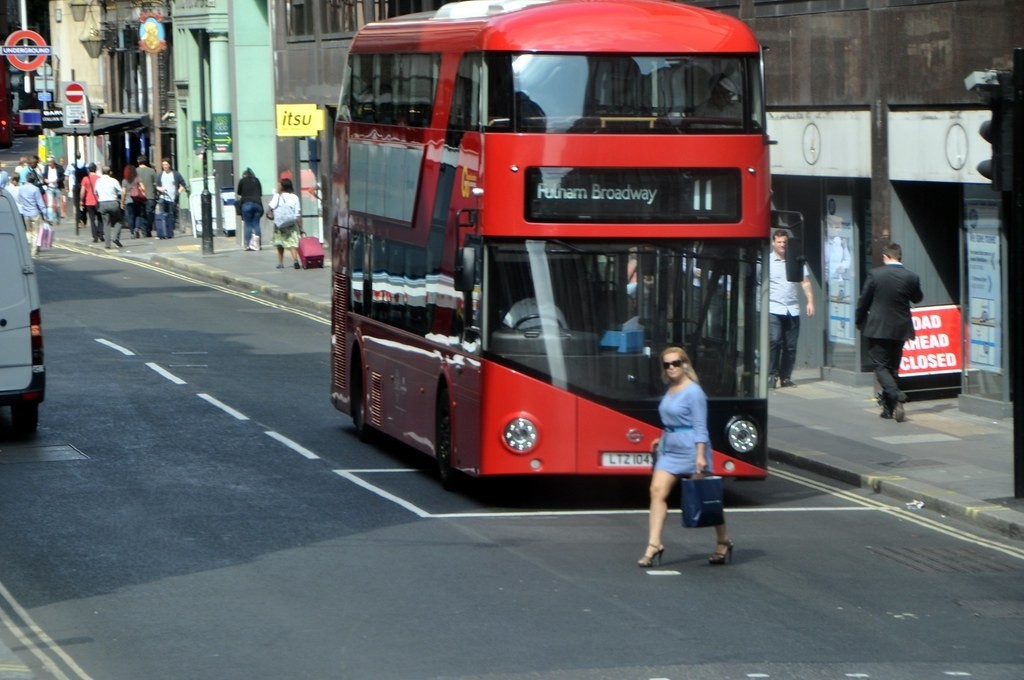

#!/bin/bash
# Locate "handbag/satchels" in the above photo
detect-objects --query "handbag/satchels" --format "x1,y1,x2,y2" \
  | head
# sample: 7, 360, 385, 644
37, 221, 55, 251
272, 204, 296, 231
267, 209, 275, 220
681, 476, 727, 527
247, 232, 263, 251
163, 200, 172, 216
234, 198, 243, 216
130, 186, 147, 204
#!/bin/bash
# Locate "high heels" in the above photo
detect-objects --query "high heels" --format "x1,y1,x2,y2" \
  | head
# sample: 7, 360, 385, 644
637, 542, 664, 567
708, 540, 735, 566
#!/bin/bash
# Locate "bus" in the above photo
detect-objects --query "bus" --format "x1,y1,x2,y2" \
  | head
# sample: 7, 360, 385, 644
320, 0, 810, 491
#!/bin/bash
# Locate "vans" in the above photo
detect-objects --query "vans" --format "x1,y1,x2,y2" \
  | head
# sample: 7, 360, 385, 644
0, 188, 50, 434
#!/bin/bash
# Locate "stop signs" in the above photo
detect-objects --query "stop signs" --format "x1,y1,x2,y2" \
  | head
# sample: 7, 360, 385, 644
62, 81, 88, 104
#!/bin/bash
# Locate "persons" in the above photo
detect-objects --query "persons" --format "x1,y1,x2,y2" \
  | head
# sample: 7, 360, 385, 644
156, 158, 186, 240
681, 248, 731, 340
502, 296, 570, 332
628, 247, 638, 283
64, 152, 122, 248
120, 156, 157, 239
855, 242, 924, 422
690, 73, 742, 129
636, 347, 733, 568
267, 178, 303, 269
238, 168, 264, 251
757, 230, 814, 388
310, 183, 322, 201
0, 155, 65, 239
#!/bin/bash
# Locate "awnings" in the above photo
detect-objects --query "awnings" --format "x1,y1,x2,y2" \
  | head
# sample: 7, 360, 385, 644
54, 118, 139, 136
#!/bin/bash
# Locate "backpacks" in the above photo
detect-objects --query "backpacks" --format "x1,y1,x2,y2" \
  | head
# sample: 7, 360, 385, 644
69, 163, 87, 189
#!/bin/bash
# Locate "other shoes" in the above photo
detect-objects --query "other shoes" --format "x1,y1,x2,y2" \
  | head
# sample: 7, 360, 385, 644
768, 376, 778, 389
155, 236, 166, 240
112, 239, 123, 249
143, 233, 152, 237
276, 264, 283, 268
781, 378, 797, 388
294, 259, 300, 270
167, 237, 172, 239
243, 247, 251, 252
134, 228, 140, 240
879, 411, 893, 419
894, 393, 908, 422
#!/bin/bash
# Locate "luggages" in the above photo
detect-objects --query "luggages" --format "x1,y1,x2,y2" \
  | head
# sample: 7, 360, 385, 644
299, 229, 326, 269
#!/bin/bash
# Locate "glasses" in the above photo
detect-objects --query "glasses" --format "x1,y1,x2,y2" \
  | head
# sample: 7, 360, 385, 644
663, 360, 684, 369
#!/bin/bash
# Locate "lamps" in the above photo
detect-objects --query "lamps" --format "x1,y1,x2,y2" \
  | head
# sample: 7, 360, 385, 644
80, 26, 104, 58
68, 0, 88, 21
